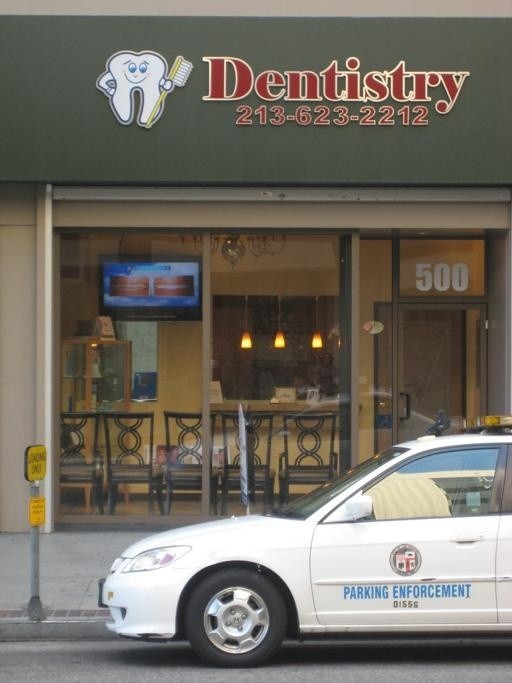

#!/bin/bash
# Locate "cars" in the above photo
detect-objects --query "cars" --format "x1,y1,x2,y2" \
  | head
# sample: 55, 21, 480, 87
99, 415, 512, 667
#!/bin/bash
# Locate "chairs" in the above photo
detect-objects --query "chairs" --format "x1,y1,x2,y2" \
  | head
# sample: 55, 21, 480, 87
59, 410, 337, 516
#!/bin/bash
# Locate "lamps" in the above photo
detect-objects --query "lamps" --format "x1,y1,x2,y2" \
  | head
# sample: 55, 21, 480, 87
182, 233, 286, 270
274, 296, 285, 349
240, 295, 253, 349
312, 297, 323, 349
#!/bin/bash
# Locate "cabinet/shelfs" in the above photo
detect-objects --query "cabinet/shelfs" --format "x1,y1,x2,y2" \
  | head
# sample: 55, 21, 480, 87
59, 336, 132, 509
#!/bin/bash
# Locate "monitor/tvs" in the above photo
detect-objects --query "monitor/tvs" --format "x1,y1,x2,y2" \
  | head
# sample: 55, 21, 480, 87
97, 253, 202, 322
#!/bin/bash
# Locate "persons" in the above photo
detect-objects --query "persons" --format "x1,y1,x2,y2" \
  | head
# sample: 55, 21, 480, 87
313, 351, 340, 398
